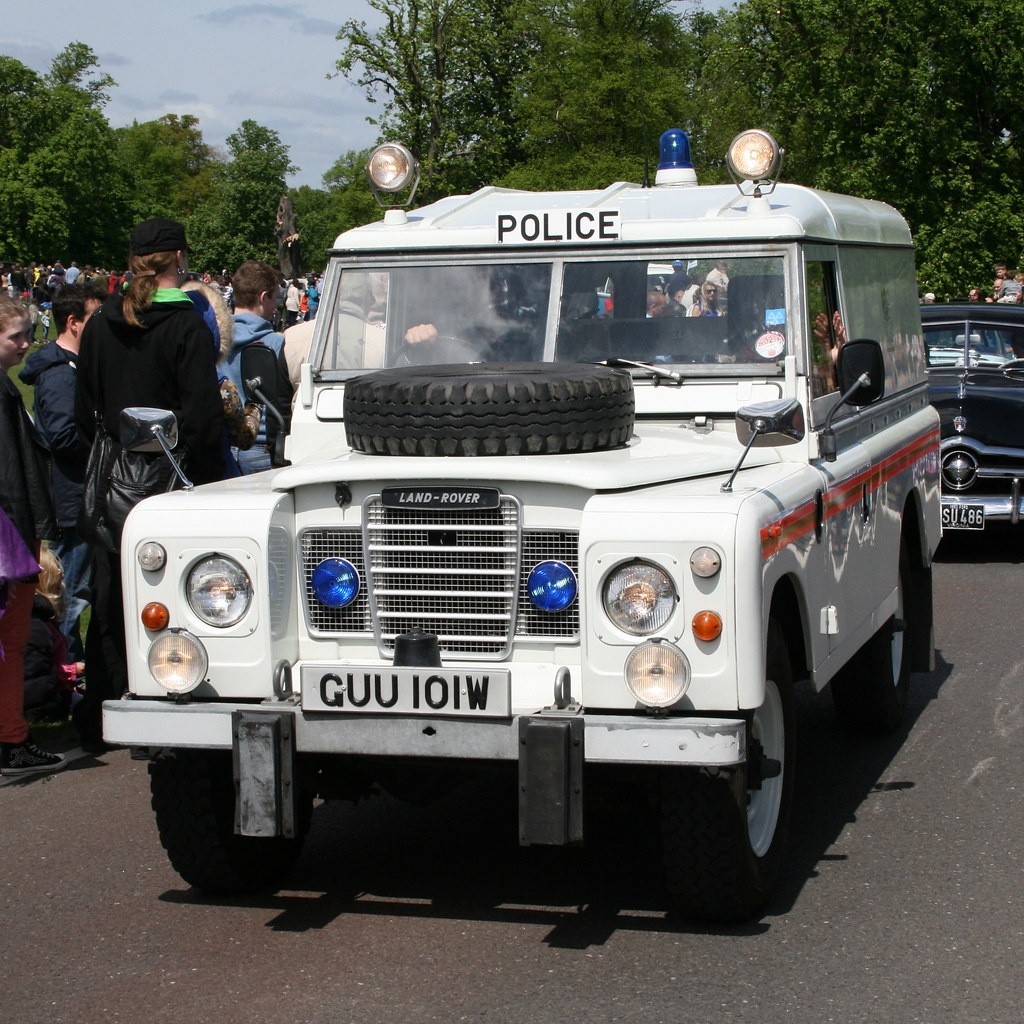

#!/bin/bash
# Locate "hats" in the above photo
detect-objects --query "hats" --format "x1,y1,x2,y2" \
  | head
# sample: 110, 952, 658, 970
131, 219, 188, 256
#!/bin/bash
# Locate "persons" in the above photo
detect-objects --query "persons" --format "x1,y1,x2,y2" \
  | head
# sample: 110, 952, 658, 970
919, 262, 1024, 367
880, 332, 925, 389
1, 218, 846, 777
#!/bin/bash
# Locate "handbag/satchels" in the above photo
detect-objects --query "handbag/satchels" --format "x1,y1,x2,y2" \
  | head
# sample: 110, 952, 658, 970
83, 430, 188, 556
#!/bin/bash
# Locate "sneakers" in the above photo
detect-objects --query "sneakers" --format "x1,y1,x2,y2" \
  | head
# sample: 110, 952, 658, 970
1, 738, 67, 776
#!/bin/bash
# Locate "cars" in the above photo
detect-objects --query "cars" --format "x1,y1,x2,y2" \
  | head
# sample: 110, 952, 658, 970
918, 303, 1024, 531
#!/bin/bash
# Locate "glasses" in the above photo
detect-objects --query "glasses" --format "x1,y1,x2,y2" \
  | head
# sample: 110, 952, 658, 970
707, 289, 717, 294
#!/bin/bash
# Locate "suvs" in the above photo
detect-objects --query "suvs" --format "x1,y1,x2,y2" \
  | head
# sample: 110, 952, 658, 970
99, 127, 946, 909
